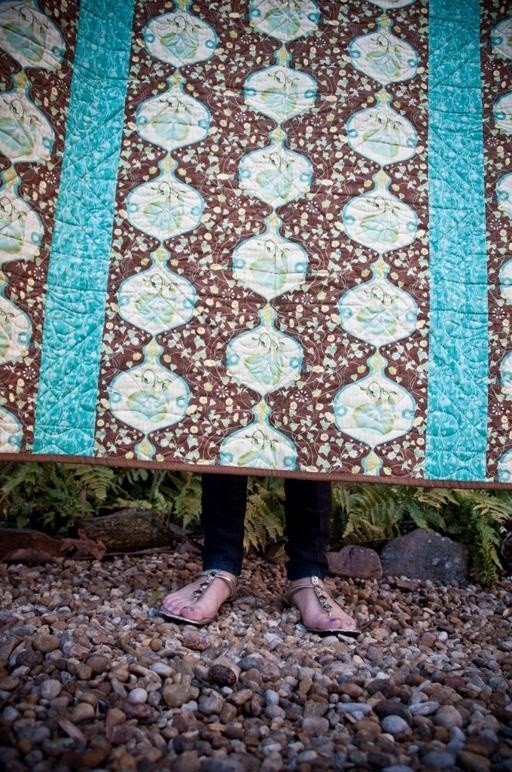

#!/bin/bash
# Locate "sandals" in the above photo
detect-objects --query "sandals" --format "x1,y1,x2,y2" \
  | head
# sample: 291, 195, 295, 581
283, 576, 362, 636
159, 568, 238, 626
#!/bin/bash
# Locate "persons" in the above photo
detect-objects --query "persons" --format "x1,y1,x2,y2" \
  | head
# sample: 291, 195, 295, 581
159, 473, 363, 635
